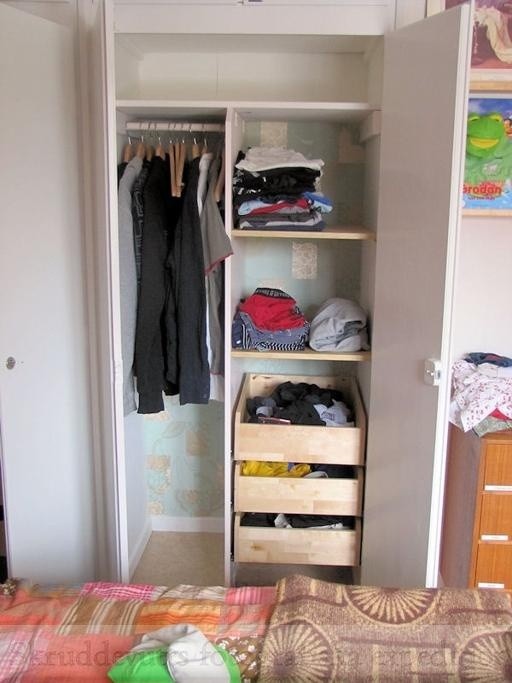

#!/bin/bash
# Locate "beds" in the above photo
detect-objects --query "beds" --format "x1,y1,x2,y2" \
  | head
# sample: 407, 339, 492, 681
0, 584, 512, 683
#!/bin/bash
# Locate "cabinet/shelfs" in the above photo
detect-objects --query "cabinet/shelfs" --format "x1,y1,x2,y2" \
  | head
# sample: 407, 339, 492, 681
75, 2, 475, 589
439, 421, 512, 590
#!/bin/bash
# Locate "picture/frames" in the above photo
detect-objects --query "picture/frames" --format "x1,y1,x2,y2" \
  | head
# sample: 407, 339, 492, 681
455, 82, 512, 216
424, 0, 512, 80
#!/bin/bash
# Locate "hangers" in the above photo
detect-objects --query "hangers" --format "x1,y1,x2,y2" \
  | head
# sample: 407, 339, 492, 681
124, 120, 225, 202
